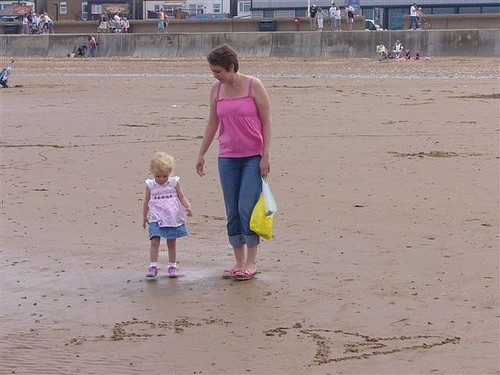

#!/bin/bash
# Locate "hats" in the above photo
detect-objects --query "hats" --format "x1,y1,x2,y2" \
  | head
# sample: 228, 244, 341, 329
164, 15, 169, 19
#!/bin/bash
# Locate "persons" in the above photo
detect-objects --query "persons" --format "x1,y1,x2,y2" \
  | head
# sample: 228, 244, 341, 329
309, 3, 430, 62
195, 45, 274, 279
141, 153, 193, 278
0, 6, 171, 87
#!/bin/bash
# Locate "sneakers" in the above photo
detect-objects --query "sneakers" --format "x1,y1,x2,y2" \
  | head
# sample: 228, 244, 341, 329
167, 265, 179, 277
145, 265, 158, 279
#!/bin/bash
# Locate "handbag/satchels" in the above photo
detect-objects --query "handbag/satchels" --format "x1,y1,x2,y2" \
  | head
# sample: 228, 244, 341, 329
261, 178, 278, 218
249, 177, 273, 241
7, 63, 12, 75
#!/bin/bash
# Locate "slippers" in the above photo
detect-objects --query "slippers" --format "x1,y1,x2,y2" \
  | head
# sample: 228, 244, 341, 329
235, 268, 258, 280
223, 267, 244, 279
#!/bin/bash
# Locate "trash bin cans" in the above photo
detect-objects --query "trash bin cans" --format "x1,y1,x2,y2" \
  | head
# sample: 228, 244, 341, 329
259, 20, 275, 31
4, 24, 19, 35
